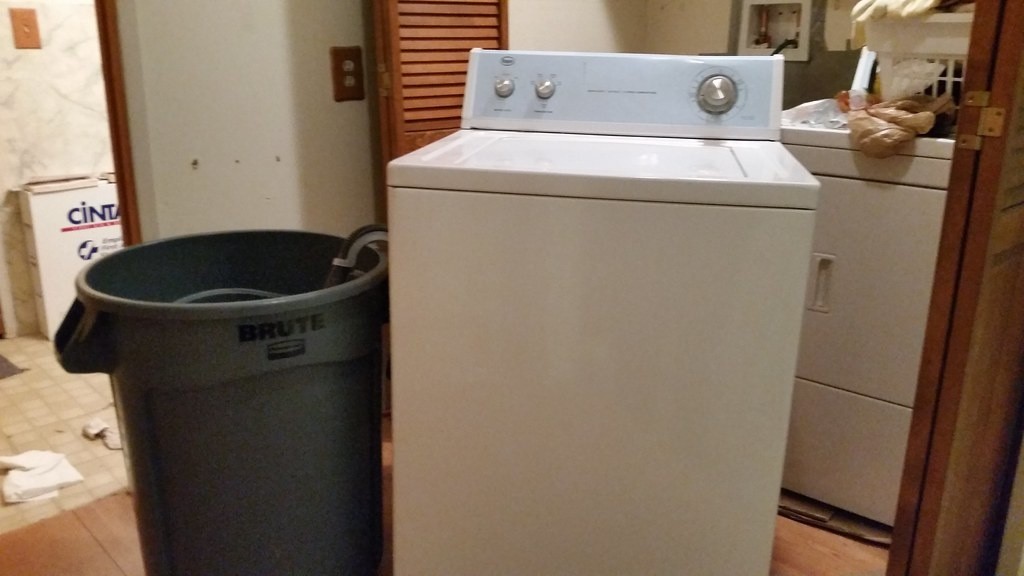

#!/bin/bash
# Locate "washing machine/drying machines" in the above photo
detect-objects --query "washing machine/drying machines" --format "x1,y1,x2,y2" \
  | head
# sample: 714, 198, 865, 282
384, 45, 822, 574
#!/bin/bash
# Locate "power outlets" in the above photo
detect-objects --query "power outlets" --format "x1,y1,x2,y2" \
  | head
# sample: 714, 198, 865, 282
329, 46, 365, 101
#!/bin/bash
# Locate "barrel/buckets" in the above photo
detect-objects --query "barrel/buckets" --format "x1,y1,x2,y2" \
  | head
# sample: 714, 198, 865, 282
55, 229, 392, 576
